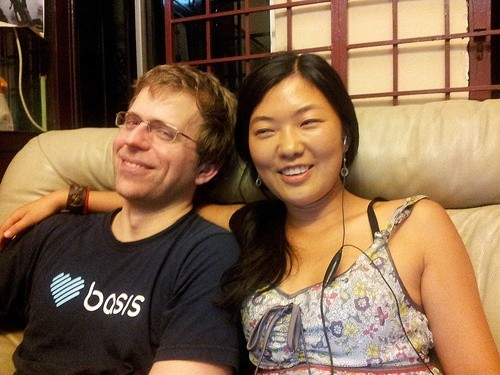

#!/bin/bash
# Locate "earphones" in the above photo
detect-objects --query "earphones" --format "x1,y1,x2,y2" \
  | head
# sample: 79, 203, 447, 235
344, 134, 348, 145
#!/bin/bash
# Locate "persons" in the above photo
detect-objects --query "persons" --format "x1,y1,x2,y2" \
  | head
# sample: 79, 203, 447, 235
0, 62, 245, 375
0, 50, 500, 375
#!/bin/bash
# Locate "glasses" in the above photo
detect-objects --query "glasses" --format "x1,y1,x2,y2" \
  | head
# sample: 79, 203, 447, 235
115, 112, 202, 147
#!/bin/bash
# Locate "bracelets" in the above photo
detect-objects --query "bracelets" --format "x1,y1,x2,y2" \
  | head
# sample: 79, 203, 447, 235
65, 182, 90, 213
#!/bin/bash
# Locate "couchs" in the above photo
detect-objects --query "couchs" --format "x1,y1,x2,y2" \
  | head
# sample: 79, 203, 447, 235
0, 98, 500, 375
